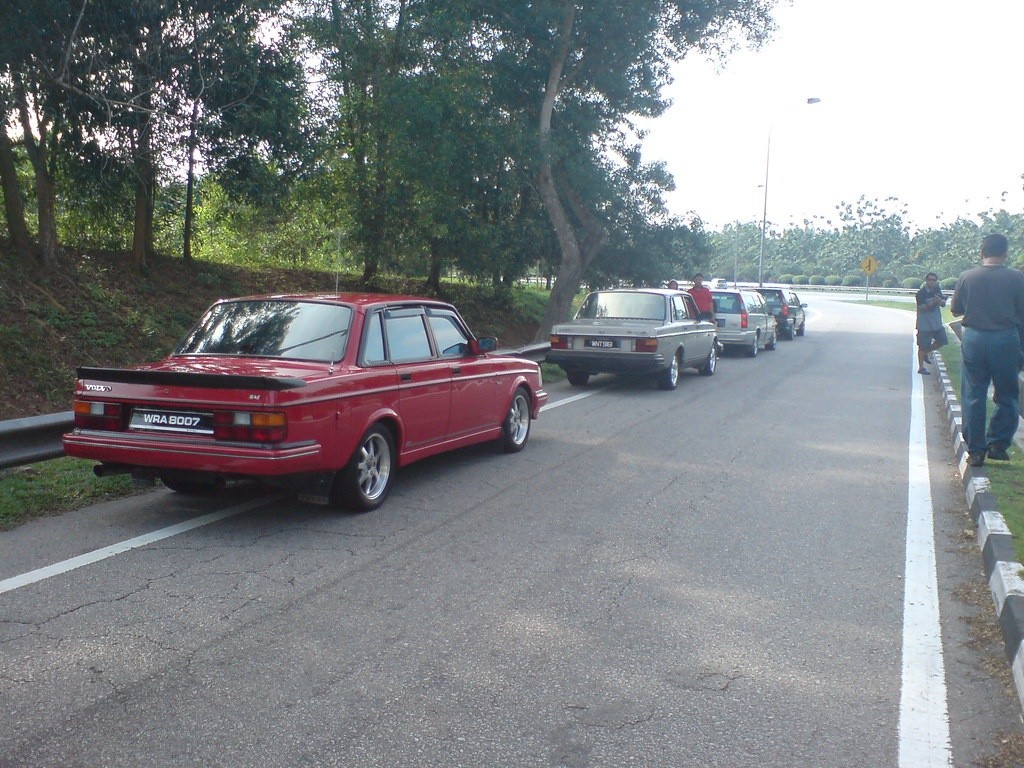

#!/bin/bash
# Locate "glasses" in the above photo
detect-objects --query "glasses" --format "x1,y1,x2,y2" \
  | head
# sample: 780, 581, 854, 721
928, 278, 936, 281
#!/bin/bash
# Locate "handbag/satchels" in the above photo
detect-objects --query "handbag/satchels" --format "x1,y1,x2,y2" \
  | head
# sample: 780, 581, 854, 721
717, 343, 723, 353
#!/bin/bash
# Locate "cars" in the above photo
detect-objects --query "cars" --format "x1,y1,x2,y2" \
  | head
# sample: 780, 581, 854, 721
707, 289, 778, 357
755, 288, 808, 340
61, 288, 544, 511
549, 286, 720, 390
709, 277, 728, 289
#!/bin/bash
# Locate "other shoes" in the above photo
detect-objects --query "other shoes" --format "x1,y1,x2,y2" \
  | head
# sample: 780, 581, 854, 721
918, 368, 930, 374
967, 453, 986, 466
924, 356, 931, 364
989, 445, 1008, 460
716, 358, 719, 360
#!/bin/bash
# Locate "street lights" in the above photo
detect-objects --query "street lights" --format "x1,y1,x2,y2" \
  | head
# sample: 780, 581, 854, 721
756, 95, 821, 281
733, 184, 764, 288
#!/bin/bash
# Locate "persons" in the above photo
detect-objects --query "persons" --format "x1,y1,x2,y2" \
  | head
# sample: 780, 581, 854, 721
668, 279, 678, 290
950, 234, 1024, 466
688, 274, 721, 361
915, 273, 948, 375
